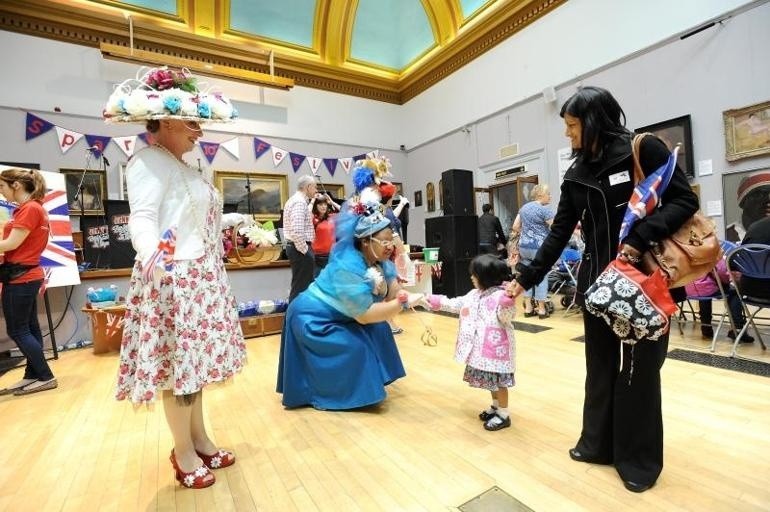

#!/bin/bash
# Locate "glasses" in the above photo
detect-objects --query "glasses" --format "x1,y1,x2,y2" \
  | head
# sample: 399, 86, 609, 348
372, 238, 396, 247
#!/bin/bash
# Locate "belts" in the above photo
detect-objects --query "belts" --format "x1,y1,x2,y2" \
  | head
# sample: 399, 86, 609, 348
287, 242, 312, 244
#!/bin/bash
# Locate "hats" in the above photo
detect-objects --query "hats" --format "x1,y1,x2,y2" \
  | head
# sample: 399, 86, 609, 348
354, 211, 391, 239
103, 67, 238, 123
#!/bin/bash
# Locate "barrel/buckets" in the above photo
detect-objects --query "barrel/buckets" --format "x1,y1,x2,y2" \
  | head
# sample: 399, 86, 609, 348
422, 246, 440, 264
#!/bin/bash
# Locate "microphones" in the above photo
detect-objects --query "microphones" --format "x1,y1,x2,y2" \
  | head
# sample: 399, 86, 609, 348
87, 145, 98, 152
246, 176, 250, 188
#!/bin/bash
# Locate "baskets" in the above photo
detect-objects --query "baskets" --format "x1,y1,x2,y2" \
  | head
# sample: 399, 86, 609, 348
227, 222, 281, 262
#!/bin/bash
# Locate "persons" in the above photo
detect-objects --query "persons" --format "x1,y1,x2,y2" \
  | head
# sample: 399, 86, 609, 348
736, 200, 769, 302
105, 64, 249, 488
513, 185, 557, 320
423, 254, 516, 430
736, 113, 769, 137
0, 167, 58, 396
278, 175, 411, 308
276, 201, 432, 411
478, 204, 507, 258
505, 87, 699, 492
684, 256, 755, 344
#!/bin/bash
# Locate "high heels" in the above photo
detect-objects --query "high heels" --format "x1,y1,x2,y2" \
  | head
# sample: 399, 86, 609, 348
170, 446, 235, 488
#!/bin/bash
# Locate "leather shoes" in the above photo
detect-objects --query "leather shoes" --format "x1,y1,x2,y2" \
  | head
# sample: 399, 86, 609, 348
728, 330, 755, 343
700, 325, 714, 337
626, 480, 648, 493
569, 448, 585, 462
0, 379, 58, 396
479, 406, 510, 431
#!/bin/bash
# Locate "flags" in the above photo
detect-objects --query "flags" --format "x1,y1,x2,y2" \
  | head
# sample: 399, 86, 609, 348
620, 142, 679, 243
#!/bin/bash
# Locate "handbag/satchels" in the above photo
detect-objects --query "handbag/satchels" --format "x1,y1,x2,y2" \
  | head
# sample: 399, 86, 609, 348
582, 258, 678, 346
0, 261, 39, 282
631, 133, 724, 289
315, 255, 328, 267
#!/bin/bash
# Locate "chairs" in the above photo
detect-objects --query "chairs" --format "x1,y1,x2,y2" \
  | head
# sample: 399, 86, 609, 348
726, 241, 770, 360
674, 266, 740, 353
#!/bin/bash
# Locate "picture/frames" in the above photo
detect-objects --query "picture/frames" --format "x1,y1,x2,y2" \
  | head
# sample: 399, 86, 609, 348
391, 181, 402, 195
414, 190, 422, 207
212, 169, 290, 221
722, 100, 770, 164
315, 183, 345, 200
59, 168, 108, 217
634, 114, 694, 177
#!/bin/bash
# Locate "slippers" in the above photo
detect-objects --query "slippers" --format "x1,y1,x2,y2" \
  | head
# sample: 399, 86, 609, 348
525, 309, 550, 319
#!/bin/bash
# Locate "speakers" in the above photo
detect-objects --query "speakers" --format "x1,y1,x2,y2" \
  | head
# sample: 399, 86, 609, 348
424, 216, 478, 297
442, 169, 474, 216
